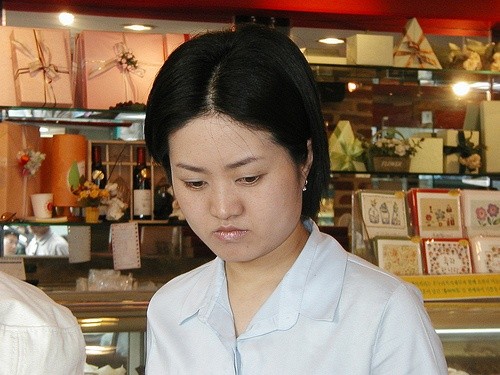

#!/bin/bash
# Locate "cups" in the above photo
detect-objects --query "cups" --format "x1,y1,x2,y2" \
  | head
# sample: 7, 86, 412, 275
315, 182, 335, 223
29, 192, 53, 221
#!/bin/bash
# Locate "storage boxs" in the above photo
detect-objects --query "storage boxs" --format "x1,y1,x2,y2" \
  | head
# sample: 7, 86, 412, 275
345, 34, 393, 64
11, 30, 73, 108
481, 101, 500, 174
411, 138, 444, 173
437, 131, 480, 172
82, 31, 164, 110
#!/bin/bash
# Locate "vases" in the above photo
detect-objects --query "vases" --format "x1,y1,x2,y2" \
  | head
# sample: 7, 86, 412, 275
31, 193, 54, 218
84, 206, 100, 223
365, 156, 411, 171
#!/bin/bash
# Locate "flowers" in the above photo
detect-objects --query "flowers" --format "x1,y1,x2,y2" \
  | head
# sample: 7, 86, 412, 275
78, 183, 106, 204
16, 148, 46, 176
363, 136, 424, 156
459, 139, 481, 169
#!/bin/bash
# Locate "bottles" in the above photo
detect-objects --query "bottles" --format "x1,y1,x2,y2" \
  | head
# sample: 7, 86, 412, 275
132, 146, 152, 220
91, 145, 109, 219
153, 175, 173, 220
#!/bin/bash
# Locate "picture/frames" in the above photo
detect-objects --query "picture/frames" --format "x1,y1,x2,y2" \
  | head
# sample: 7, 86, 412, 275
358, 189, 499, 275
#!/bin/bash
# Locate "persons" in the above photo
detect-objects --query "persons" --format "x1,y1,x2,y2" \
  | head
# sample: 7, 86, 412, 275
2, 223, 69, 256
144, 26, 447, 375
